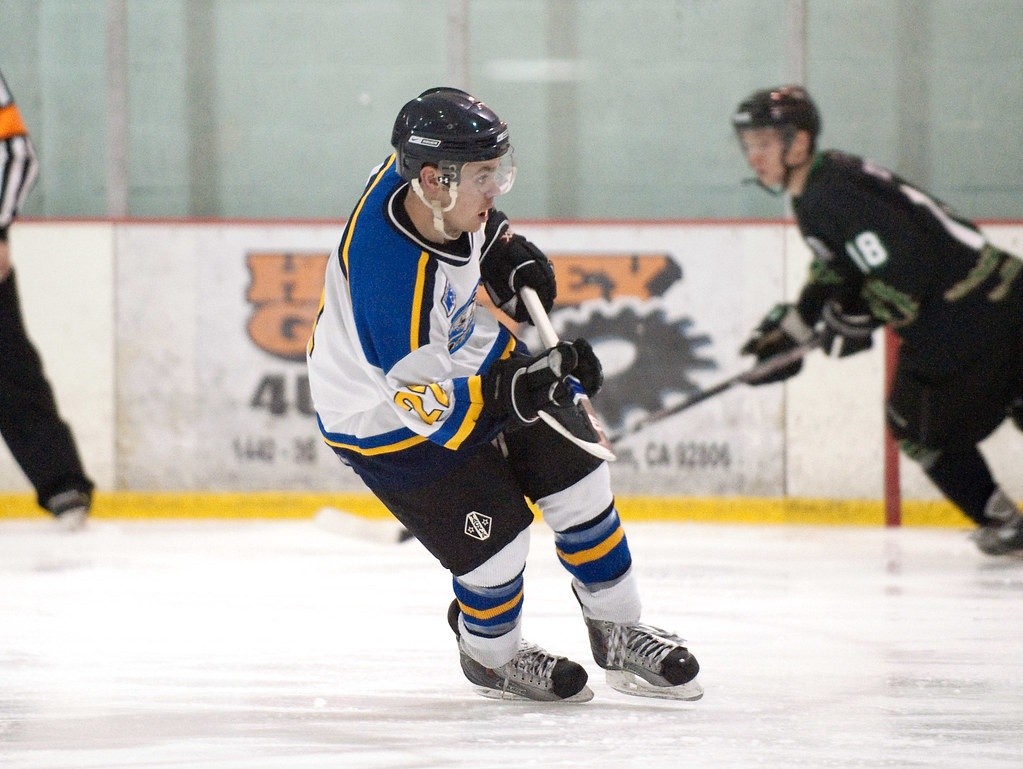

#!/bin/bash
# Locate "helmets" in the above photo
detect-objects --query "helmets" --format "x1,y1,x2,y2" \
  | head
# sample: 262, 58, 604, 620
733, 84, 819, 134
392, 87, 517, 198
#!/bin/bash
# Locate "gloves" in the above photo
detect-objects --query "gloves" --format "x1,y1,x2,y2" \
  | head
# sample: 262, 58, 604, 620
820, 302, 873, 358
741, 303, 813, 385
487, 339, 604, 426
479, 206, 557, 325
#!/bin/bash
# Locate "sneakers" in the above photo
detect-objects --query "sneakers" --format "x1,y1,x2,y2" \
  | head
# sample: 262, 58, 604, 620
571, 579, 704, 702
448, 598, 594, 704
977, 486, 1023, 559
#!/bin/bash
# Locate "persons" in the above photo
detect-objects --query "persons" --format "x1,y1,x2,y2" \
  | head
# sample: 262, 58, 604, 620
731, 83, 1022, 556
0, 73, 94, 532
306, 88, 704, 702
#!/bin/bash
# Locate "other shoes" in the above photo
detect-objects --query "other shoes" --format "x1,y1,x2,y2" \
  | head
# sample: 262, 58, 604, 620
55, 506, 87, 535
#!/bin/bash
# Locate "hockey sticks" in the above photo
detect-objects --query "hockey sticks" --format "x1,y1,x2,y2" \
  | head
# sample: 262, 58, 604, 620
518, 286, 622, 467
310, 333, 827, 550
